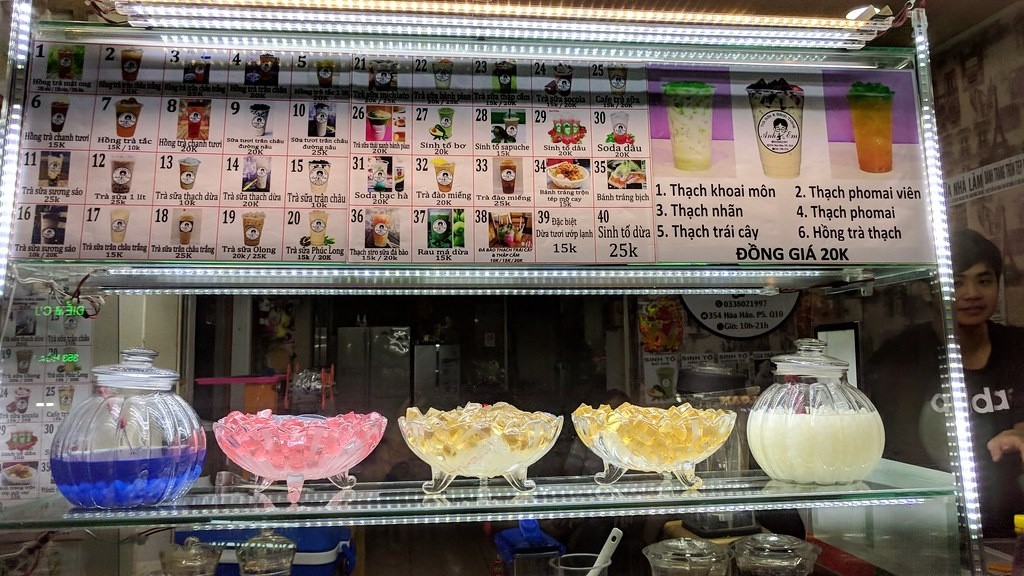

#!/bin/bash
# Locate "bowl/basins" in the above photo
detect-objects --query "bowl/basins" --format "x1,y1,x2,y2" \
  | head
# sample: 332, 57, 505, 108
548, 164, 589, 188
573, 404, 738, 489
3, 467, 36, 483
398, 412, 564, 492
214, 412, 387, 502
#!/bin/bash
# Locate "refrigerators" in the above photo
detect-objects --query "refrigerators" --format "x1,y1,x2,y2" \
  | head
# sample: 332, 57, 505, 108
336, 327, 411, 462
414, 345, 460, 411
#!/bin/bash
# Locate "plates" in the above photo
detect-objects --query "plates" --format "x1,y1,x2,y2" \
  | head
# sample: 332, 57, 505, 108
7, 437, 37, 450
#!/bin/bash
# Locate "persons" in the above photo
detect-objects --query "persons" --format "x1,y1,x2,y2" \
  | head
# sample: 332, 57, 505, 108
870, 228, 1024, 540
557, 387, 634, 555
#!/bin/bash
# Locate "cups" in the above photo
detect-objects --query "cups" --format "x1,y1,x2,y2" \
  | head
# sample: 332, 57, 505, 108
188, 102, 204, 138
20, 310, 34, 333
259, 55, 274, 80
112, 155, 134, 193
315, 107, 328, 136
440, 110, 454, 137
372, 160, 389, 191
500, 166, 516, 193
59, 387, 74, 410
64, 349, 77, 372
121, 49, 143, 80
662, 84, 717, 170
15, 390, 31, 413
309, 163, 330, 192
608, 66, 628, 94
40, 212, 59, 244
497, 65, 512, 90
58, 50, 75, 79
16, 350, 32, 373
848, 96, 893, 173
374, 214, 390, 246
196, 61, 206, 80
250, 108, 270, 136
554, 72, 572, 96
110, 210, 130, 242
243, 213, 264, 246
513, 547, 560, 576
433, 62, 453, 89
257, 159, 268, 188
374, 61, 393, 90
179, 215, 194, 244
436, 163, 455, 191
504, 118, 519, 130
431, 208, 450, 240
115, 103, 142, 137
48, 155, 63, 185
51, 102, 69, 132
549, 553, 611, 576
309, 213, 330, 245
610, 114, 628, 144
178, 160, 200, 189
65, 312, 78, 336
747, 89, 804, 178
367, 112, 391, 141
318, 58, 335, 87
